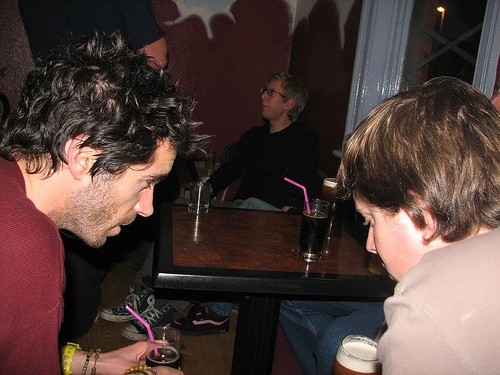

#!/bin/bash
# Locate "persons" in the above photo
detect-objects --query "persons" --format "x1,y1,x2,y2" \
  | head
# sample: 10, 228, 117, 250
17, 0, 168, 344
334, 75, 500, 375
172, 197, 305, 338
278, 87, 500, 375
97, 71, 318, 342
0, 29, 217, 375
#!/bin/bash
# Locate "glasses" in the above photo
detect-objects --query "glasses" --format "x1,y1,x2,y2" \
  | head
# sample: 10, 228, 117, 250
259, 88, 286, 99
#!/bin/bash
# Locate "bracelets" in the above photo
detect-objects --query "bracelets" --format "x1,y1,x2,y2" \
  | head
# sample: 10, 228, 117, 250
91, 348, 102, 375
81, 348, 94, 375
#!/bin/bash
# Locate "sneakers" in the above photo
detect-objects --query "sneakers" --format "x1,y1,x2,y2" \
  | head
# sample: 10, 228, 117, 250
121, 300, 175, 341
100, 285, 156, 322
171, 303, 230, 336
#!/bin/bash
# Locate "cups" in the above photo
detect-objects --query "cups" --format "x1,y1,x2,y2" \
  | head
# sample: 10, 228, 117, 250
187, 181, 211, 214
296, 198, 331, 264
205, 148, 215, 177
146, 326, 182, 370
322, 178, 344, 240
331, 333, 382, 375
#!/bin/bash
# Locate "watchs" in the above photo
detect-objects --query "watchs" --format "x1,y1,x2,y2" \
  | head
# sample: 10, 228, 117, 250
61, 342, 81, 375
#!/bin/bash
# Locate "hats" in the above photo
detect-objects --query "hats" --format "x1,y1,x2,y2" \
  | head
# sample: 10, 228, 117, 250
17, 0, 167, 60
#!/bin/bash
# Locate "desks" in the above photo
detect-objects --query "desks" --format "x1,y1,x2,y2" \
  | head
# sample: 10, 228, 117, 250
152, 203, 397, 375
